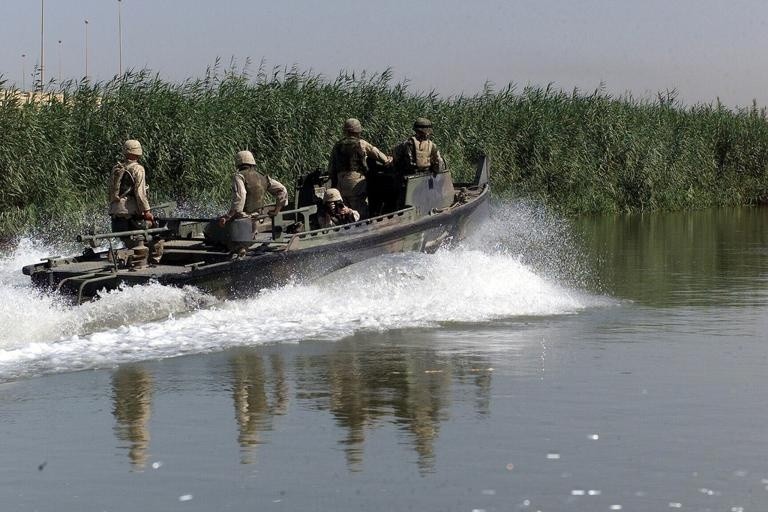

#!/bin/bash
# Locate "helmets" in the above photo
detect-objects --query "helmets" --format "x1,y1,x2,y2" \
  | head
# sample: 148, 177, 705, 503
123, 139, 143, 156
322, 188, 344, 205
343, 117, 363, 134
234, 151, 257, 167
412, 117, 434, 136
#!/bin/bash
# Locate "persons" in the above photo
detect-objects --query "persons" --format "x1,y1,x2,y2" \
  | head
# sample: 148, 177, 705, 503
313, 187, 359, 231
202, 150, 289, 239
390, 116, 442, 214
106, 139, 157, 247
326, 117, 393, 220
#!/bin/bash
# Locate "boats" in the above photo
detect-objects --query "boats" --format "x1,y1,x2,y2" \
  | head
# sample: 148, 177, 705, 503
21, 154, 490, 311
